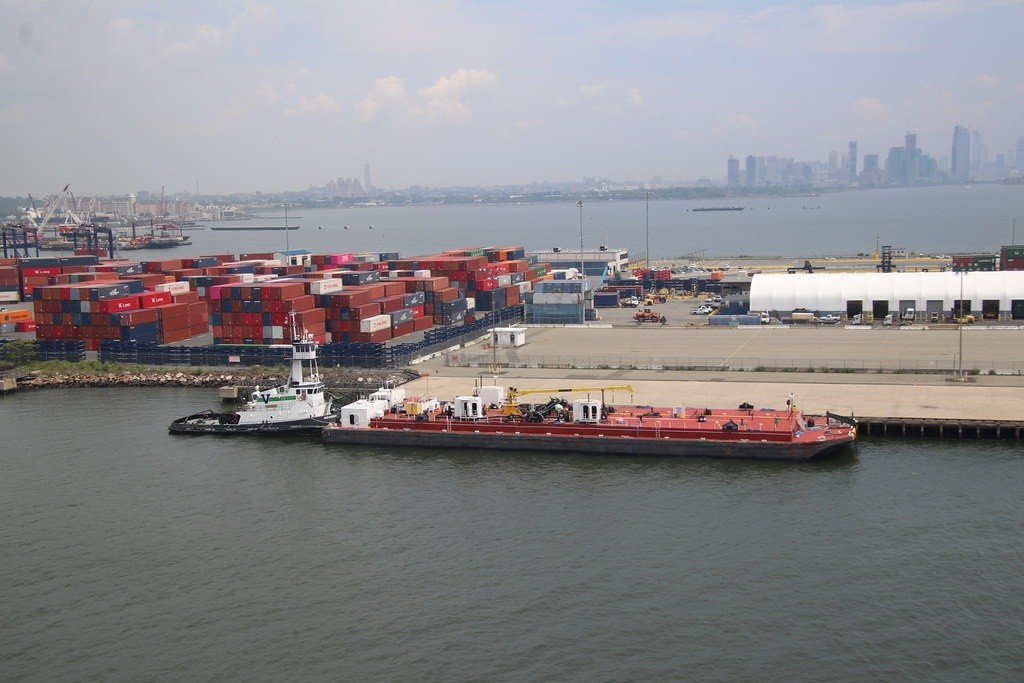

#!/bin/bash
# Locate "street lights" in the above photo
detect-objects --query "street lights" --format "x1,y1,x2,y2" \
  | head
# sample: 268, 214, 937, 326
576, 200, 585, 284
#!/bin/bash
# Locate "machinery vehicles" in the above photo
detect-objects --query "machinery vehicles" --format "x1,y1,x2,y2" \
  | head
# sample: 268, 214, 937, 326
634, 307, 661, 323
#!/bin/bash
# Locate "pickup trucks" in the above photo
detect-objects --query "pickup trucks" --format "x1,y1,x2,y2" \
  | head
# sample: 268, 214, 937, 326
691, 305, 713, 315
820, 314, 842, 322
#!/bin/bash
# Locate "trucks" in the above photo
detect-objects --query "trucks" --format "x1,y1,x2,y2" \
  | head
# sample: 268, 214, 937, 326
747, 311, 770, 325
851, 311, 873, 326
907, 307, 915, 320
618, 296, 639, 307
883, 315, 912, 326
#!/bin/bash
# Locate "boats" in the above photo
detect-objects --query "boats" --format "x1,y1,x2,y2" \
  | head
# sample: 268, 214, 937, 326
319, 379, 859, 460
234, 308, 338, 427
